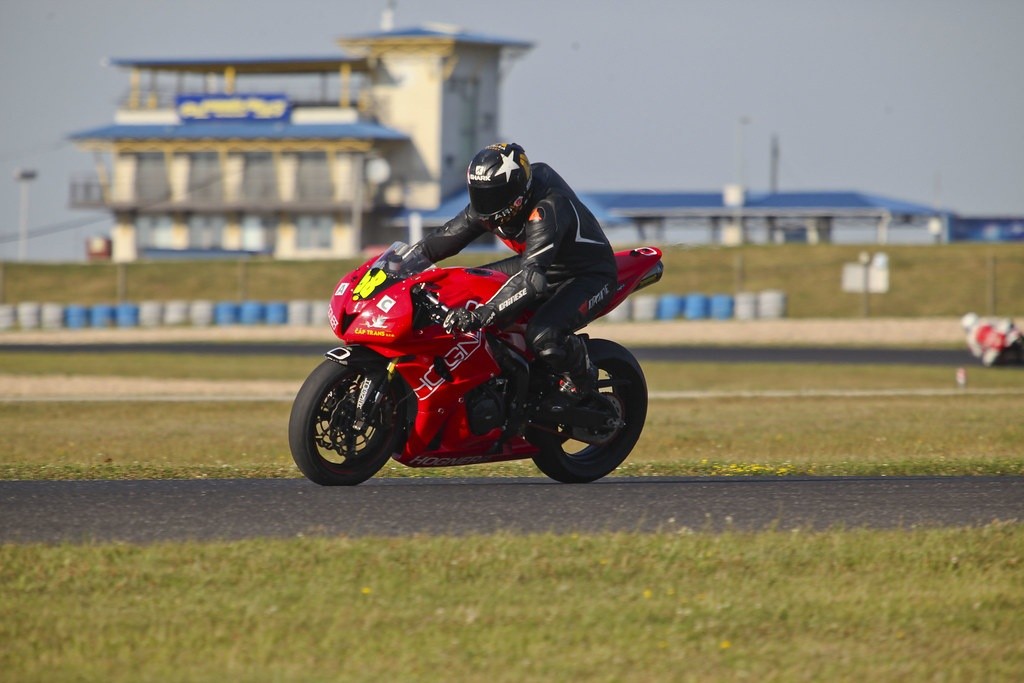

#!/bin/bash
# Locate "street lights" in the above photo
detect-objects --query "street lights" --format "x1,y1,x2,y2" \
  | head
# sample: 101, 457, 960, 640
14, 168, 37, 261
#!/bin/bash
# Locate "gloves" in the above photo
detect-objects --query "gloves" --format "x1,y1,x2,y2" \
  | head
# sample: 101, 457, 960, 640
443, 308, 480, 334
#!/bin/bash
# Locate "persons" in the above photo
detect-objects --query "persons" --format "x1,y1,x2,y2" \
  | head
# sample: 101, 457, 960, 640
963, 314, 1024, 368
387, 143, 619, 406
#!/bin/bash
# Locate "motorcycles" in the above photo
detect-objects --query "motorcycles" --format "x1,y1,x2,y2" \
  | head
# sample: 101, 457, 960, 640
289, 245, 663, 486
972, 319, 1024, 369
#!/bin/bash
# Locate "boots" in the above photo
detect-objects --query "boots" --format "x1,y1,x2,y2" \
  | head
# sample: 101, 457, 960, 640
569, 336, 597, 406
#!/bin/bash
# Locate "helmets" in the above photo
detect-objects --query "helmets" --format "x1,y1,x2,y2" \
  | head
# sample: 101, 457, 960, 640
468, 143, 533, 230
